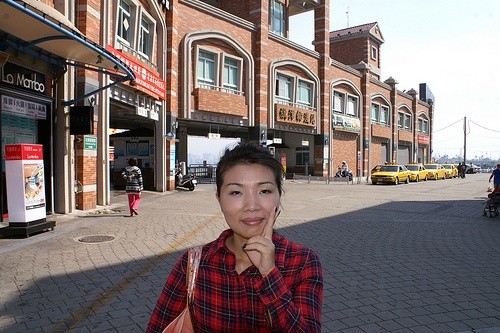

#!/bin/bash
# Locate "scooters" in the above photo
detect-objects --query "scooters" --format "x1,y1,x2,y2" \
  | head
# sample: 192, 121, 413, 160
334, 165, 352, 181
175, 164, 198, 191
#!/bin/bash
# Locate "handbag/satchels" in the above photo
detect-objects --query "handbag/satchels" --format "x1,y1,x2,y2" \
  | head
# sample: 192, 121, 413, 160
485, 182, 492, 192
162, 246, 203, 333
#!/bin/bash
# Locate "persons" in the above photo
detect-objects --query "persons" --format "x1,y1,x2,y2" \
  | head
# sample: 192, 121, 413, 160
145, 142, 323, 333
470, 162, 473, 174
340, 161, 348, 177
457, 162, 465, 179
489, 163, 500, 193
122, 157, 143, 216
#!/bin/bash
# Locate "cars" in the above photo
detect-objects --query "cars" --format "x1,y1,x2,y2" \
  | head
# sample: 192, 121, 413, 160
465, 164, 481, 173
371, 164, 411, 184
424, 164, 448, 180
405, 164, 428, 182
442, 164, 458, 178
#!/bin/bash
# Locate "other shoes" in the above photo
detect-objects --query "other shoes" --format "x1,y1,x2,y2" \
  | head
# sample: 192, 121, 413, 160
129, 208, 139, 216
482, 213, 486, 216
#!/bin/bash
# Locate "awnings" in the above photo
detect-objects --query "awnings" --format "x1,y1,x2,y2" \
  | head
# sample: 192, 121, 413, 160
0, 0, 136, 106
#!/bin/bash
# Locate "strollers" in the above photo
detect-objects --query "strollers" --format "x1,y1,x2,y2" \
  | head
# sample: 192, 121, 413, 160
482, 191, 500, 218
